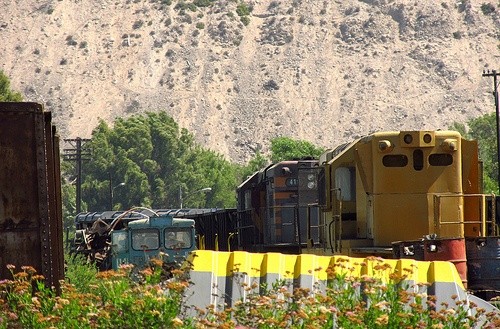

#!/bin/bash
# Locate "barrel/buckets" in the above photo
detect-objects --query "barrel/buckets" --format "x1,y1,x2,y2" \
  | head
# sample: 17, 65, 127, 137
424, 238, 467, 291
392, 241, 425, 261
464, 237, 500, 291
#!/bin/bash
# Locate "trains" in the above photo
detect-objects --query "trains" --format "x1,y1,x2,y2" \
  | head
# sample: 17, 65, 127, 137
75, 130, 484, 258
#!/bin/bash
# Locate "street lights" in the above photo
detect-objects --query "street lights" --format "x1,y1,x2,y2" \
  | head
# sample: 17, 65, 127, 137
111, 183, 126, 206
180, 185, 212, 209
